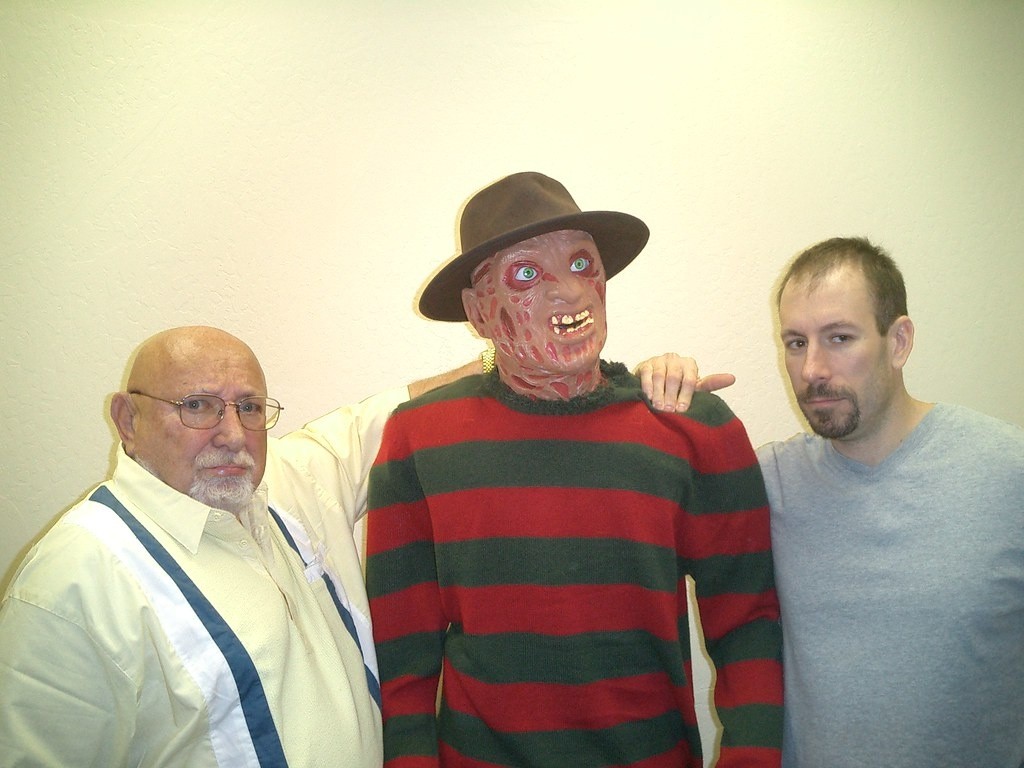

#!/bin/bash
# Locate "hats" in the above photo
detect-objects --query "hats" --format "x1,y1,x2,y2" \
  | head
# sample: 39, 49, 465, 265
419, 172, 651, 323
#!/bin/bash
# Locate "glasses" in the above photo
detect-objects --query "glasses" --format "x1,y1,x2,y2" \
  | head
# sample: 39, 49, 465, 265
130, 391, 285, 432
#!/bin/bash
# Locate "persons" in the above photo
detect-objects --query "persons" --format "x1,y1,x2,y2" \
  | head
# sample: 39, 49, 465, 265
0, 326, 496, 768
363, 170, 784, 768
632, 238, 1024, 768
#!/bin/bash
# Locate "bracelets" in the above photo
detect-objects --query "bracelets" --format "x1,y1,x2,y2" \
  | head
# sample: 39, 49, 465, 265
481, 348, 496, 374
